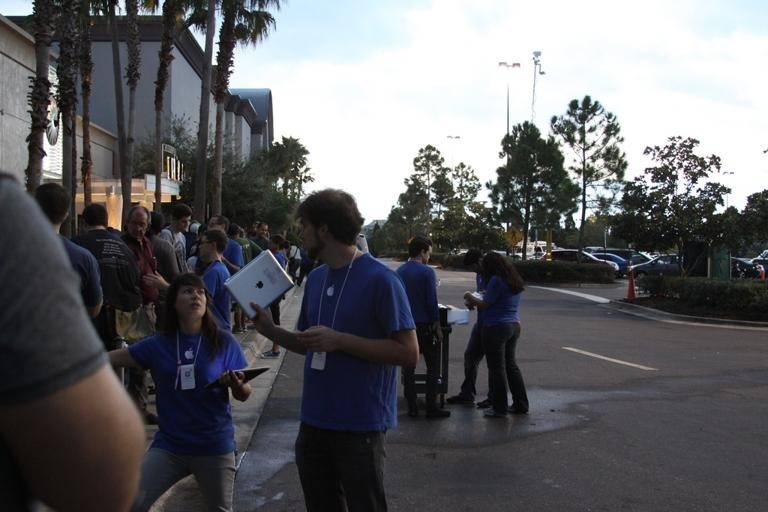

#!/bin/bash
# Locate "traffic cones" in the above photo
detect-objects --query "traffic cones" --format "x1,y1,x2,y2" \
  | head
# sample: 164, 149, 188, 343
625, 268, 638, 301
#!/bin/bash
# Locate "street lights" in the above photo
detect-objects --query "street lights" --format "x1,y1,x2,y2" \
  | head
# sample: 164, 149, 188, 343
528, 50, 546, 123
447, 135, 461, 185
497, 61, 521, 256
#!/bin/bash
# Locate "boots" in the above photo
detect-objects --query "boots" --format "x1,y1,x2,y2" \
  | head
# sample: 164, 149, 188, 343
406, 393, 451, 420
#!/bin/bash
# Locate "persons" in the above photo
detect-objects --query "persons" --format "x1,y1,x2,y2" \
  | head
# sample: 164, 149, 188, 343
462, 250, 530, 418
247, 188, 420, 511
150, 203, 270, 334
444, 247, 509, 407
262, 234, 288, 358
731, 261, 747, 279
286, 241, 302, 282
395, 233, 451, 419
70, 203, 138, 351
119, 205, 171, 418
108, 272, 253, 511
0, 172, 147, 512
36, 183, 104, 319
751, 259, 766, 279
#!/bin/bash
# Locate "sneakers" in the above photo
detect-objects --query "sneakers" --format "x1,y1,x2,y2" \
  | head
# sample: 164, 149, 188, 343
261, 350, 281, 359
231, 325, 247, 334
446, 394, 528, 416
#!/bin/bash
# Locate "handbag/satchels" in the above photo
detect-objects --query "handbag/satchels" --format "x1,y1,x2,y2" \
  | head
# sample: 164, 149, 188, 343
105, 303, 155, 344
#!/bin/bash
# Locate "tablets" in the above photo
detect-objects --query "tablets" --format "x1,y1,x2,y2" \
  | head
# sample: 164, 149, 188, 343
225, 250, 295, 319
204, 366, 269, 388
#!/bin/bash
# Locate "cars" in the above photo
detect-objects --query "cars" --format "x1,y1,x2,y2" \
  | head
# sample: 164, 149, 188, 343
503, 241, 768, 283
539, 249, 619, 273
